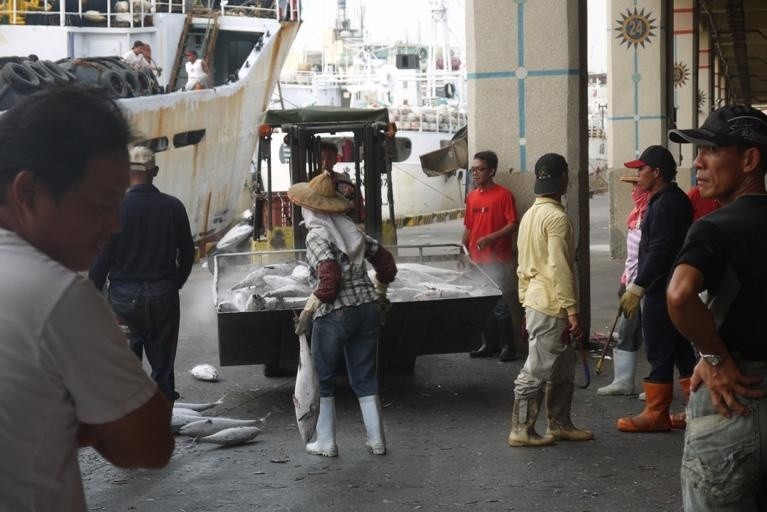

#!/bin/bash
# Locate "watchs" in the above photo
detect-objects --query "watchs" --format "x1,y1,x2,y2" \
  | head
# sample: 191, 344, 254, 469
697, 346, 726, 367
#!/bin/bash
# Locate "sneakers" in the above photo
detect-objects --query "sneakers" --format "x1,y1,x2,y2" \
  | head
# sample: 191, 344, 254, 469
470, 344, 494, 359
498, 344, 516, 362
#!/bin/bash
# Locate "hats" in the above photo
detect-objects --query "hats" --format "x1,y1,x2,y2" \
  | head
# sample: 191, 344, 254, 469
534, 153, 568, 195
131, 40, 144, 50
128, 145, 155, 172
619, 175, 641, 184
667, 104, 767, 147
624, 145, 675, 170
286, 170, 351, 214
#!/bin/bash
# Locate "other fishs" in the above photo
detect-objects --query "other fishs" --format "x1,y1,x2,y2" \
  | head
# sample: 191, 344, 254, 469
292, 309, 321, 445
170, 393, 272, 447
190, 363, 218, 382
367, 261, 477, 302
219, 259, 315, 314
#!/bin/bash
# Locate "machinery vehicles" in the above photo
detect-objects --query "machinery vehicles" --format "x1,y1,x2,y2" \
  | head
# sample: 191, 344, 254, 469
207, 102, 513, 382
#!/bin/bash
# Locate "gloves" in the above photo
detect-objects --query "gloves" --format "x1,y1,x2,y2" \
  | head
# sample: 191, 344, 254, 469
618, 282, 645, 321
293, 291, 322, 338
373, 277, 392, 315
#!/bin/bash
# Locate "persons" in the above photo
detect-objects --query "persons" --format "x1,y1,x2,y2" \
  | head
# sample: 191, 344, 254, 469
458, 150, 527, 361
306, 142, 366, 220
87, 144, 197, 406
596, 173, 656, 395
286, 168, 396, 460
505, 152, 596, 449
182, 49, 213, 90
665, 107, 766, 511
615, 144, 694, 434
122, 40, 163, 76
687, 184, 722, 223
0, 84, 175, 511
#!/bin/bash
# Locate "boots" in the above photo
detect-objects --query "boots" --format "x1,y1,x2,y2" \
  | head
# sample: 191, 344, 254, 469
595, 347, 635, 397
615, 375, 674, 433
507, 381, 555, 448
668, 375, 693, 430
357, 393, 386, 455
543, 380, 594, 442
305, 394, 338, 458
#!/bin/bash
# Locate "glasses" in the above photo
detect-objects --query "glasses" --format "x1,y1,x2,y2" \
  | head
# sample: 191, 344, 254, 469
470, 167, 488, 174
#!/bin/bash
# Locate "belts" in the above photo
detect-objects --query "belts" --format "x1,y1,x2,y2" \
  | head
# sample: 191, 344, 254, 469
729, 350, 766, 363
